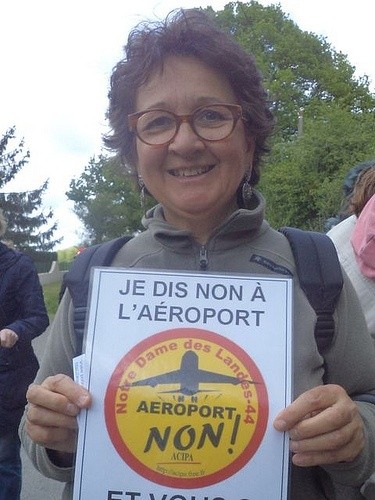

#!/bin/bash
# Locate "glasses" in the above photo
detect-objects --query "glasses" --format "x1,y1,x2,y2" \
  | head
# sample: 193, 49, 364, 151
126, 103, 250, 147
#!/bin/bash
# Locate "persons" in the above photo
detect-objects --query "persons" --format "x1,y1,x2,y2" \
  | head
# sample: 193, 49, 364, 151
0, 198, 51, 500
17, 7, 375, 500
324, 161, 373, 338
